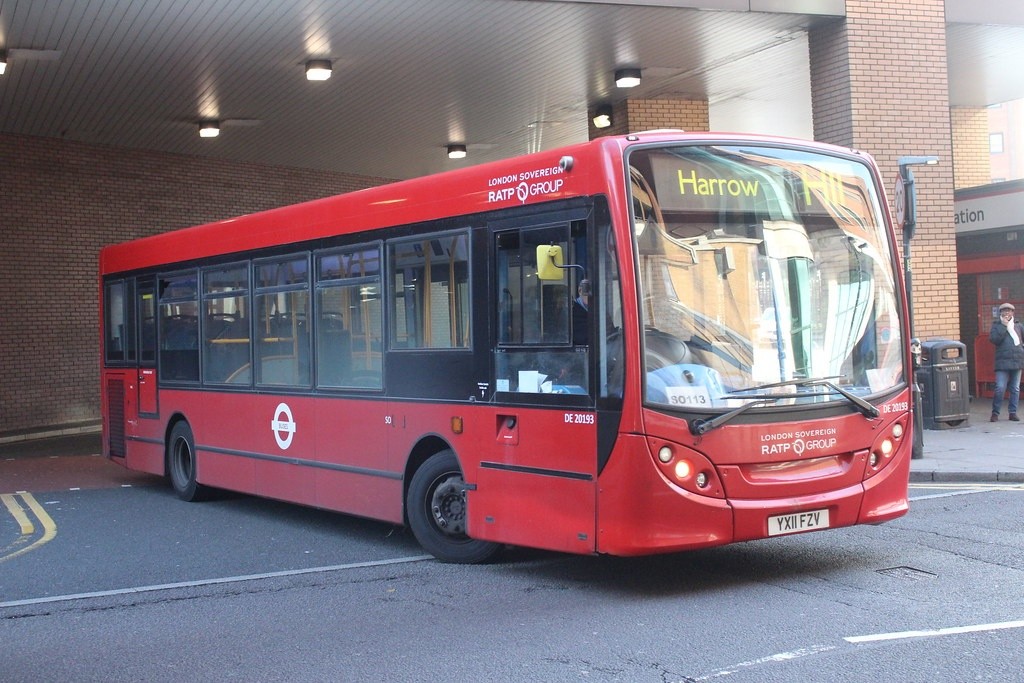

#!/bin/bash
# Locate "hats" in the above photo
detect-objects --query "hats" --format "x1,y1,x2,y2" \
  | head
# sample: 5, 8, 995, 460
999, 303, 1015, 311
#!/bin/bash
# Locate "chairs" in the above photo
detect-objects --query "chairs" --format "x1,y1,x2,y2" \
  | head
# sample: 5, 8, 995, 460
117, 309, 344, 387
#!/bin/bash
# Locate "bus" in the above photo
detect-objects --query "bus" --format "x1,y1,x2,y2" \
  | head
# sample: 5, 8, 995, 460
98, 128, 913, 563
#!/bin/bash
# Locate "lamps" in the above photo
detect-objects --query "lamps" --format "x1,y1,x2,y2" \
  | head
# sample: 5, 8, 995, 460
305, 59, 332, 82
615, 67, 641, 88
448, 144, 466, 159
198, 120, 220, 138
593, 104, 613, 129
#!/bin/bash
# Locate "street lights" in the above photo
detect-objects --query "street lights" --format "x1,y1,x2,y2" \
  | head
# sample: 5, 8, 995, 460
898, 155, 939, 460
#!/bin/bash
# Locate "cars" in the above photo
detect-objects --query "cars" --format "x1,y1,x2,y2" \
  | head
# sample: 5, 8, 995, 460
757, 307, 778, 347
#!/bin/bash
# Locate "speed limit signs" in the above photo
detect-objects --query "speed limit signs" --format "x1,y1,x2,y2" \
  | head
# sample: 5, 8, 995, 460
894, 173, 905, 229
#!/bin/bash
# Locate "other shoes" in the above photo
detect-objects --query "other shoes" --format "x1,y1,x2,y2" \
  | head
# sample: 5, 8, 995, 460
991, 415, 998, 422
1009, 413, 1019, 421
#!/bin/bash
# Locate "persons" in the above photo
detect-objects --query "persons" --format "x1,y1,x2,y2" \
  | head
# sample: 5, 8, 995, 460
536, 278, 619, 346
989, 303, 1024, 422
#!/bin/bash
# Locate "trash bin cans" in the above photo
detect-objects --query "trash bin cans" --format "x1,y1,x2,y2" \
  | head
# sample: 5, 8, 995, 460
919, 338, 971, 430
